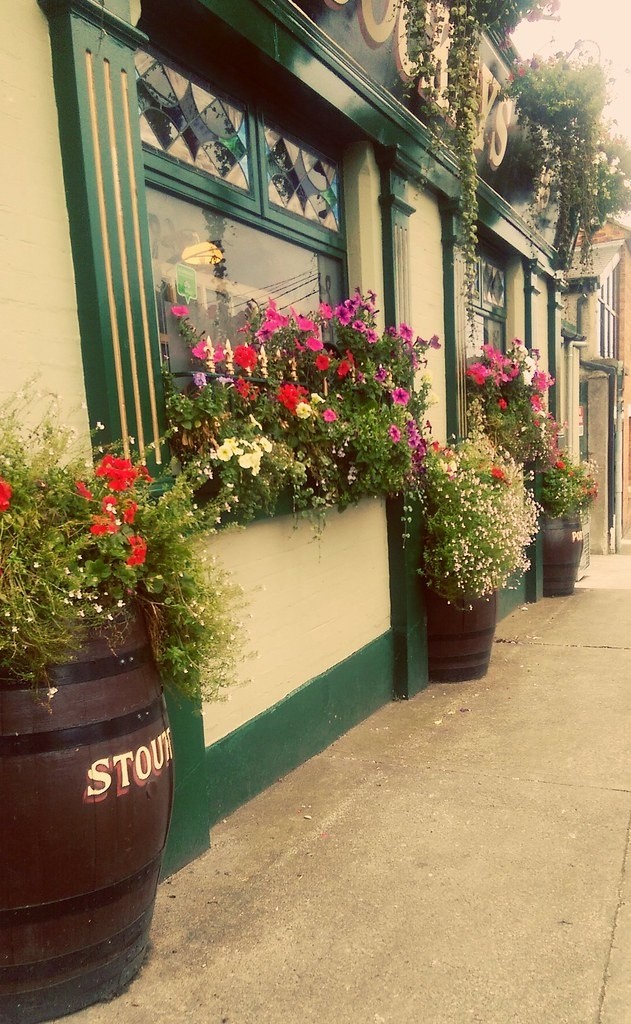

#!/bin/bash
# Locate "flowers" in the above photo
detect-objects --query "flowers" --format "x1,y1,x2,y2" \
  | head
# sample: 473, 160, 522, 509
0, 373, 255, 714
543, 453, 601, 519
463, 342, 568, 472
394, 0, 630, 351
155, 287, 438, 545
421, 425, 545, 610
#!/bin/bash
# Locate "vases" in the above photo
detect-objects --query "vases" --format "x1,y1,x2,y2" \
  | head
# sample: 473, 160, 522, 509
544, 517, 585, 595
427, 584, 498, 683
0, 587, 174, 1023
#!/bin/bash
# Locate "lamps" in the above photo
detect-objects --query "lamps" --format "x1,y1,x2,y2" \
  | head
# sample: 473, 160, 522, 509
180, 229, 223, 266
